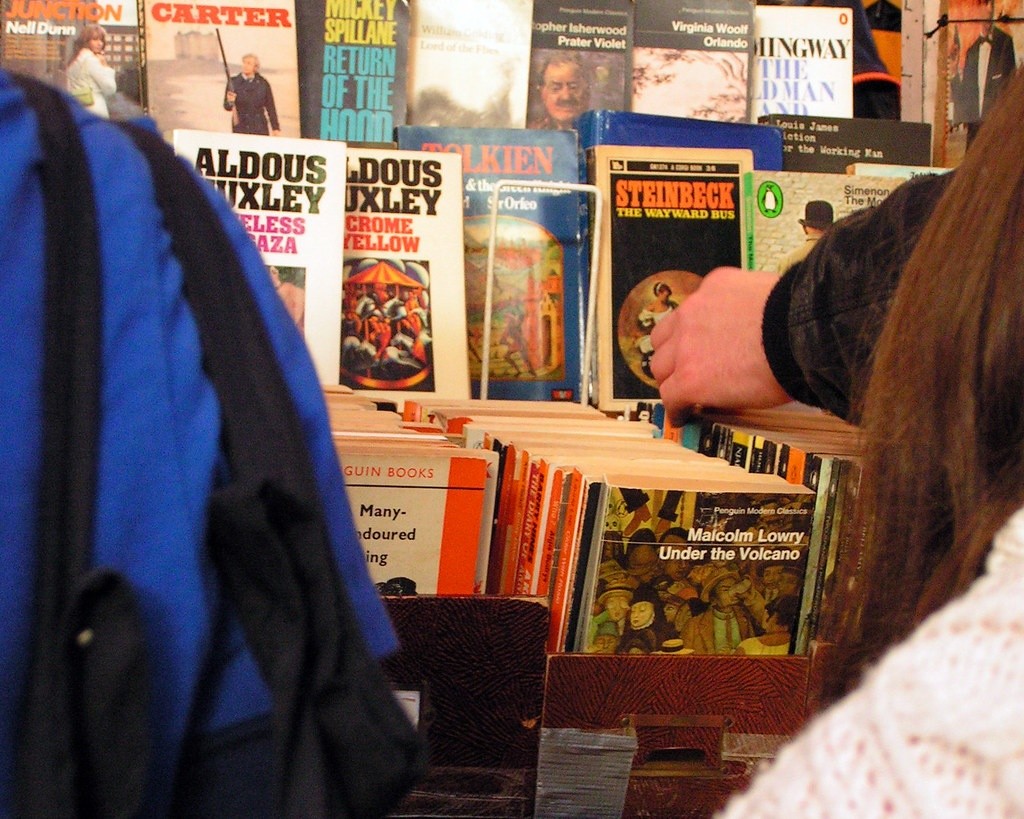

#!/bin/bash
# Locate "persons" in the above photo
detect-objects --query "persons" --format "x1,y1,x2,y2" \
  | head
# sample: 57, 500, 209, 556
223, 52, 282, 140
0, 78, 424, 819
67, 23, 118, 122
536, 50, 590, 132
714, 60, 1024, 819
647, 175, 962, 414
948, 0, 1016, 123
